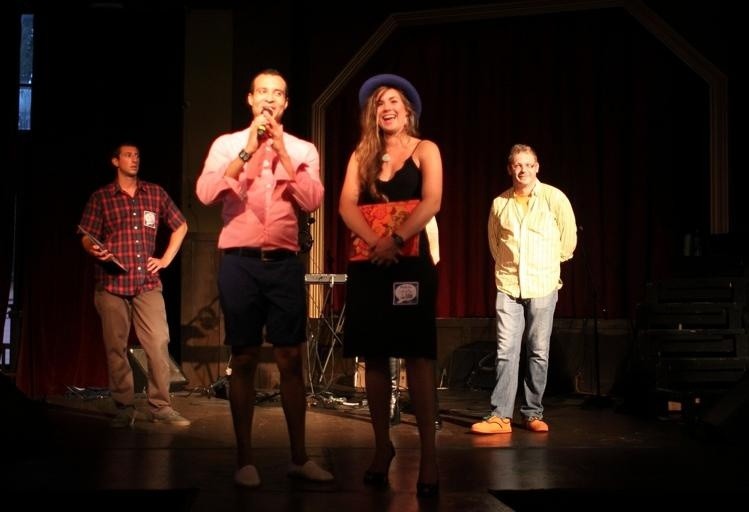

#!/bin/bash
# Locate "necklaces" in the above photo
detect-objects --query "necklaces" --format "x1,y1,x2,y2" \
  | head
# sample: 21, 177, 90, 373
381, 135, 409, 162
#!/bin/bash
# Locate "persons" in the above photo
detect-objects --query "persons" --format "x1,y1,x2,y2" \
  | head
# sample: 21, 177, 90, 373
389, 213, 441, 430
73, 140, 192, 429
469, 142, 578, 437
339, 71, 444, 503
195, 66, 334, 488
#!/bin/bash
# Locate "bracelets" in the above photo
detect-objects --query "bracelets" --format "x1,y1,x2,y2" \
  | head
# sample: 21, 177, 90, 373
239, 148, 252, 163
392, 231, 404, 248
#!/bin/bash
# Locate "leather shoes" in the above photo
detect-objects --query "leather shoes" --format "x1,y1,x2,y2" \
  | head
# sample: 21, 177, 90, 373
522, 418, 549, 432
417, 480, 440, 499
470, 416, 512, 433
363, 440, 395, 483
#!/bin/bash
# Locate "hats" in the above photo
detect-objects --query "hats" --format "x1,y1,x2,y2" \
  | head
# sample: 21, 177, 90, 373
358, 74, 422, 121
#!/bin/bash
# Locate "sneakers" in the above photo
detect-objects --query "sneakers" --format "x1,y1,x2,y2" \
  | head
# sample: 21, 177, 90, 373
148, 406, 191, 427
110, 407, 135, 427
233, 464, 261, 487
287, 459, 334, 481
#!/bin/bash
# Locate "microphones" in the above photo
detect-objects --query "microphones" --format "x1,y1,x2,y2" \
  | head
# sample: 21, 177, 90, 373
258, 105, 272, 138
